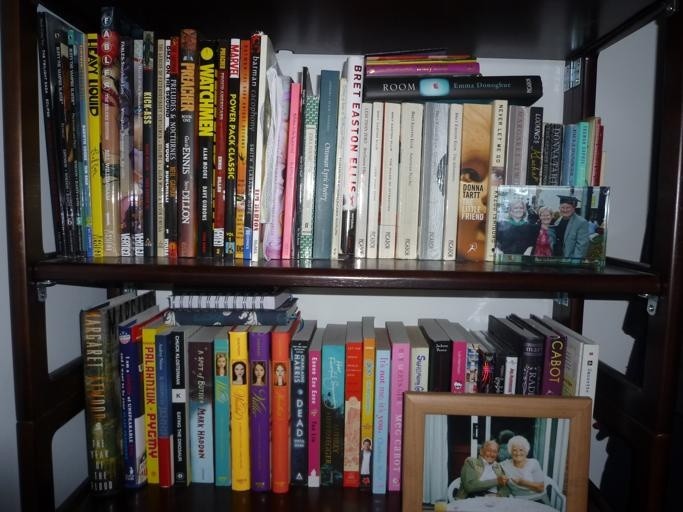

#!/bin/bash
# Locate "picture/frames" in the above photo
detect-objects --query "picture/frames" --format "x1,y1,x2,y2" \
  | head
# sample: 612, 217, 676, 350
400, 391, 592, 512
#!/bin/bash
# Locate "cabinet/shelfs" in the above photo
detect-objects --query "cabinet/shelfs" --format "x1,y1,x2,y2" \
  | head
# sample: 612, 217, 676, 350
0, 0, 682, 512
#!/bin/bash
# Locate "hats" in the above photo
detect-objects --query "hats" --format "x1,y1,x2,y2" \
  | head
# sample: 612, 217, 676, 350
556, 194, 581, 208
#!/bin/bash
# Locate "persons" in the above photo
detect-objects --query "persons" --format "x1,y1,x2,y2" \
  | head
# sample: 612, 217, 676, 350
455, 441, 509, 500
497, 434, 544, 504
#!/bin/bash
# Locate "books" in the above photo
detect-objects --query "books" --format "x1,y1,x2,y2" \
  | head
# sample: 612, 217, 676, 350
77, 288, 640, 493
34, 8, 602, 265
